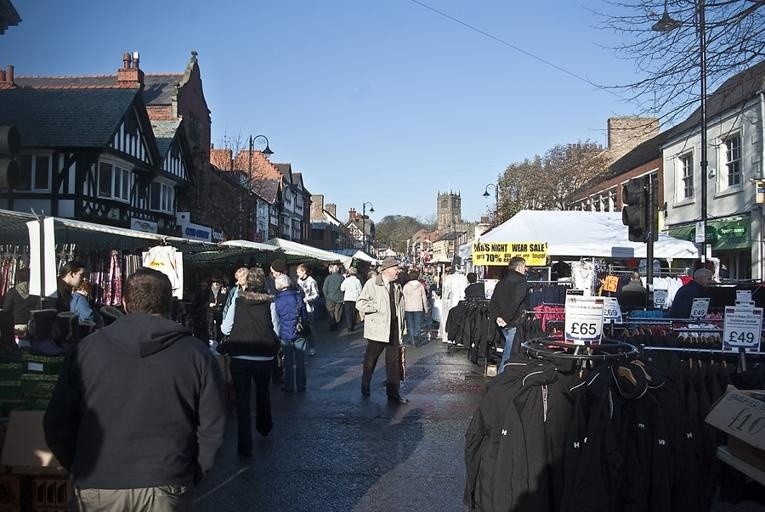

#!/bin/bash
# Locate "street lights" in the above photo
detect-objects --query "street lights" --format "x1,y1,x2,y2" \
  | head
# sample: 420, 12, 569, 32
483, 182, 500, 226
245, 134, 275, 242
361, 201, 374, 249
652, 1, 709, 263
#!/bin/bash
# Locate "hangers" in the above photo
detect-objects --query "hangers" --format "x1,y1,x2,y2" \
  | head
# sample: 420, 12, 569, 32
0, 243, 177, 265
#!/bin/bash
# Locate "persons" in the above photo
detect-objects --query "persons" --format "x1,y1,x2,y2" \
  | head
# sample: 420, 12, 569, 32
0, 254, 715, 511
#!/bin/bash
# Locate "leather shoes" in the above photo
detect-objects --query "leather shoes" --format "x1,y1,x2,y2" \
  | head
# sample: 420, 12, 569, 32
389, 395, 408, 404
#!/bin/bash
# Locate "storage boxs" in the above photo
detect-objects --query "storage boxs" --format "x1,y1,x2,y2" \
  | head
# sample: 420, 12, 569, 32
705, 385, 765, 473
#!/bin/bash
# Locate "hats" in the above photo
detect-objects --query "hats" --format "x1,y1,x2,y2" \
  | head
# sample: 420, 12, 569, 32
272, 258, 288, 274
381, 256, 400, 270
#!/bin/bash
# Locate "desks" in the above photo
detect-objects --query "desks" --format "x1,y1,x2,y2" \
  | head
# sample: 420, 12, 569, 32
716, 446, 765, 506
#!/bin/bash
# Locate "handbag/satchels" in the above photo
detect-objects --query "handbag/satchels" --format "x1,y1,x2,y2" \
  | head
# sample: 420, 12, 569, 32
293, 336, 312, 353
216, 335, 231, 356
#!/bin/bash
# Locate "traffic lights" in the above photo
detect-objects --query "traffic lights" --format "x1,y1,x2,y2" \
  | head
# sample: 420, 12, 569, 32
622, 176, 655, 243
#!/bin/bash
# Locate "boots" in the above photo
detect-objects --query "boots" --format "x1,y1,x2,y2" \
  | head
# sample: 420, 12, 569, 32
0, 306, 124, 365
